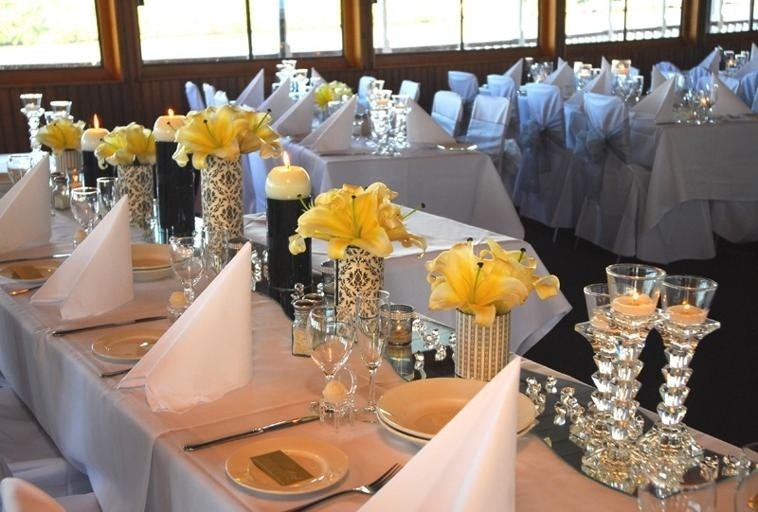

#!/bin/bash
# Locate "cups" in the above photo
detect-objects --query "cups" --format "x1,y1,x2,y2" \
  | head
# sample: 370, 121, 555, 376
582, 283, 612, 331
381, 303, 415, 347
21, 94, 42, 111
67, 168, 87, 203
96, 178, 120, 215
49, 101, 73, 118
276, 59, 410, 117
733, 440, 758, 511
637, 457, 718, 511
385, 343, 414, 380
605, 262, 667, 317
320, 261, 335, 298
660, 275, 719, 323
70, 187, 102, 234
6, 155, 32, 185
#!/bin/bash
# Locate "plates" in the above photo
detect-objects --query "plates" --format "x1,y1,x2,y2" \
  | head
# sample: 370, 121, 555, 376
1, 260, 60, 283
376, 376, 540, 447
223, 434, 349, 495
128, 244, 190, 283
93, 327, 169, 360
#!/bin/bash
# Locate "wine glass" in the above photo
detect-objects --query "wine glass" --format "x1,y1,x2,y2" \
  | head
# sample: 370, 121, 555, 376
524, 50, 753, 126
356, 291, 390, 424
168, 237, 204, 318
303, 305, 355, 414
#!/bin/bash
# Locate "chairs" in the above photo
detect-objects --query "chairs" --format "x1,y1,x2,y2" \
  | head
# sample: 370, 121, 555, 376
186, 46, 758, 275
0, 474, 70, 512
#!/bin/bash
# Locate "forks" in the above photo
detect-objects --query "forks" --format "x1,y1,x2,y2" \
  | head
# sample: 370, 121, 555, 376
283, 463, 403, 512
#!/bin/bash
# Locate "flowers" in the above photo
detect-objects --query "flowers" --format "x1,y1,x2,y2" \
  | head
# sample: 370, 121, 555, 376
35, 112, 81, 148
90, 122, 158, 165
169, 106, 280, 167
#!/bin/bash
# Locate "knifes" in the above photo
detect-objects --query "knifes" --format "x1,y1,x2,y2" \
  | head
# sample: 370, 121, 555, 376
0, 254, 72, 264
183, 415, 321, 452
52, 316, 167, 335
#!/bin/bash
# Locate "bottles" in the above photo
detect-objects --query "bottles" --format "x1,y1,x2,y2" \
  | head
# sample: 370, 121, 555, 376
50, 172, 71, 210
291, 293, 326, 357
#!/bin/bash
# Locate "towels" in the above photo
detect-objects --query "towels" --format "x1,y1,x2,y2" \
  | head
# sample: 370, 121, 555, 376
356, 353, 521, 510
0, 156, 57, 253
114, 239, 255, 412
29, 190, 134, 320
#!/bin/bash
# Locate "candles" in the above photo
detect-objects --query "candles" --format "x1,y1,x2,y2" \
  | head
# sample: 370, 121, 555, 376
151, 110, 187, 142
80, 117, 111, 150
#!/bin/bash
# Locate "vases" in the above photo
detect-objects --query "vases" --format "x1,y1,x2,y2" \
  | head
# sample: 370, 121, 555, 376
116, 164, 153, 237
453, 308, 512, 383
51, 149, 80, 172
